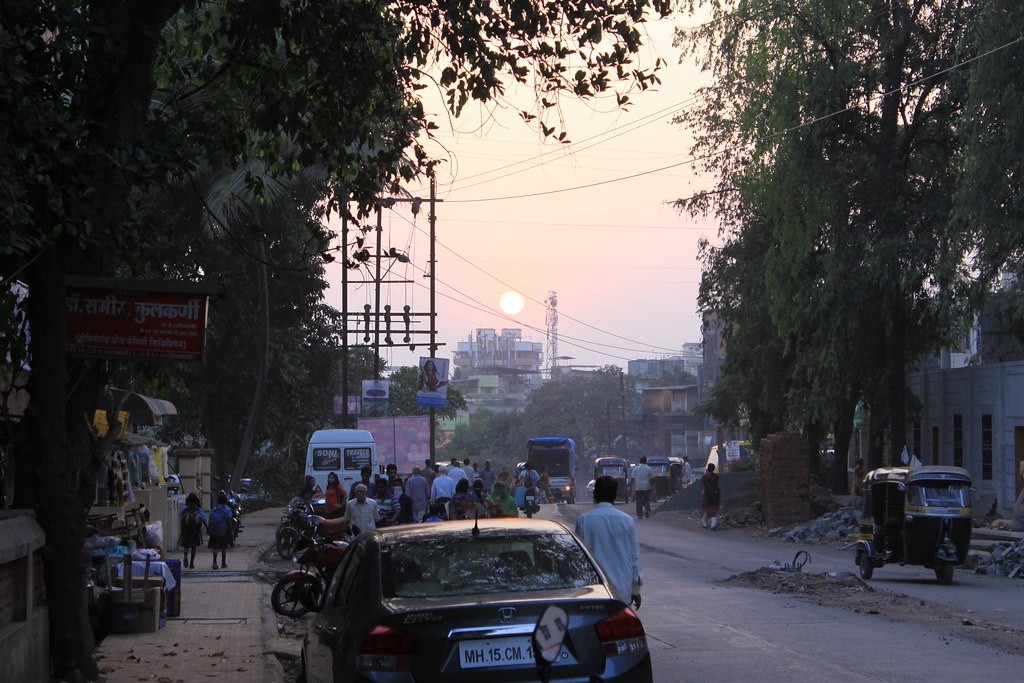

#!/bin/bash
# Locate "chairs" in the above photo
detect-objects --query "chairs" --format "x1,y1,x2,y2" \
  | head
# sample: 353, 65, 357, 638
382, 560, 434, 595
493, 549, 534, 584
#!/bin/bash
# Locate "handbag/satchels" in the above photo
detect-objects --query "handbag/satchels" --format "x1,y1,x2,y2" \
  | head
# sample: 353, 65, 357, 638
514, 485, 528, 509
524, 472, 532, 487
83, 520, 163, 560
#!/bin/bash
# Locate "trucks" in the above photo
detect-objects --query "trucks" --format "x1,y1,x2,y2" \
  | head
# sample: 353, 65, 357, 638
528, 438, 578, 504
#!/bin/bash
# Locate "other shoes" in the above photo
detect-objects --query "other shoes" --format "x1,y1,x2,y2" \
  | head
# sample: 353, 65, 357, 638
645, 510, 649, 517
221, 563, 228, 568
183, 560, 188, 568
710, 523, 718, 530
701, 519, 710, 528
211, 564, 219, 569
190, 563, 195, 569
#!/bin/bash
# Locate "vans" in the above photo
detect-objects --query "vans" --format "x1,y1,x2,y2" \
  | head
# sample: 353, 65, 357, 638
304, 428, 386, 502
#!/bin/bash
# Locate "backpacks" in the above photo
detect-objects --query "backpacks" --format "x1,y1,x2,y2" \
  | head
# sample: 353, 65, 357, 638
208, 504, 229, 538
177, 506, 204, 544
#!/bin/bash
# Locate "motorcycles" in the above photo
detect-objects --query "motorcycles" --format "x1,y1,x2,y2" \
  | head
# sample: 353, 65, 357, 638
271, 494, 352, 620
645, 456, 685, 501
854, 467, 975, 586
592, 456, 631, 504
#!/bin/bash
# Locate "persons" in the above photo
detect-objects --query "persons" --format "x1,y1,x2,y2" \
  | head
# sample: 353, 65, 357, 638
632, 457, 653, 518
346, 484, 379, 542
178, 492, 208, 569
302, 476, 321, 495
702, 463, 718, 529
349, 458, 553, 526
627, 460, 633, 497
207, 497, 234, 570
684, 456, 692, 478
325, 474, 348, 515
577, 478, 643, 611
854, 459, 865, 498
420, 360, 440, 392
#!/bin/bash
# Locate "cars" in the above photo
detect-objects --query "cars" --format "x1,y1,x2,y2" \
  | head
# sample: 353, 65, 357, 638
234, 478, 265, 504
299, 517, 654, 683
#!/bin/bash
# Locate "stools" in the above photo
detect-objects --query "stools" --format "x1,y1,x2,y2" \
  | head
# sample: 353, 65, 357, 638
105, 553, 153, 603
